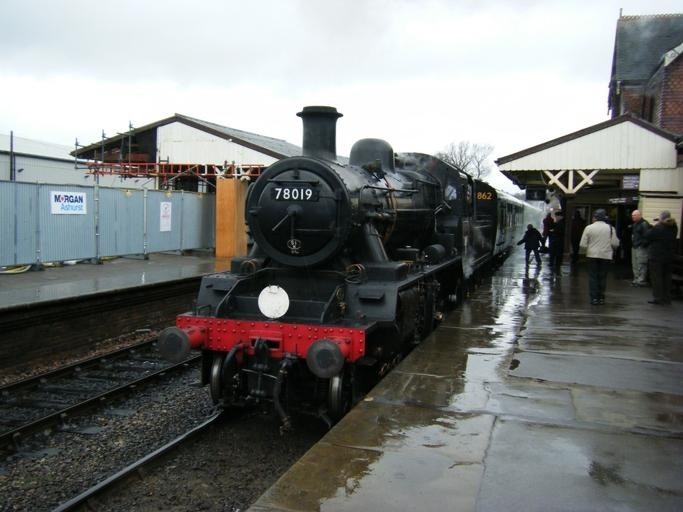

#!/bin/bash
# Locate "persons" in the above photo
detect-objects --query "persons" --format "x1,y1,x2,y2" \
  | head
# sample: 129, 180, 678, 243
541, 213, 554, 248
515, 223, 545, 267
628, 209, 653, 289
568, 210, 587, 259
545, 211, 566, 278
640, 207, 678, 305
578, 207, 620, 305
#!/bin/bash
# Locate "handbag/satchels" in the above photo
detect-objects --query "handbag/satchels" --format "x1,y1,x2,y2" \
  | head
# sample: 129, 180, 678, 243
537, 247, 551, 252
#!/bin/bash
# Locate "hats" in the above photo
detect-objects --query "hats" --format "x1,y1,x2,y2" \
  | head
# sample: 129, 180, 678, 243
654, 211, 670, 220
592, 209, 608, 221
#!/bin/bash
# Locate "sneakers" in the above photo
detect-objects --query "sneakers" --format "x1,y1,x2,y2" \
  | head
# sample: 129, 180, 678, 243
647, 298, 671, 304
591, 299, 604, 304
631, 281, 649, 287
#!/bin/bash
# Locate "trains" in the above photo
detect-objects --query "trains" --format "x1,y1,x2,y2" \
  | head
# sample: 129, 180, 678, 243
158, 99, 543, 452
